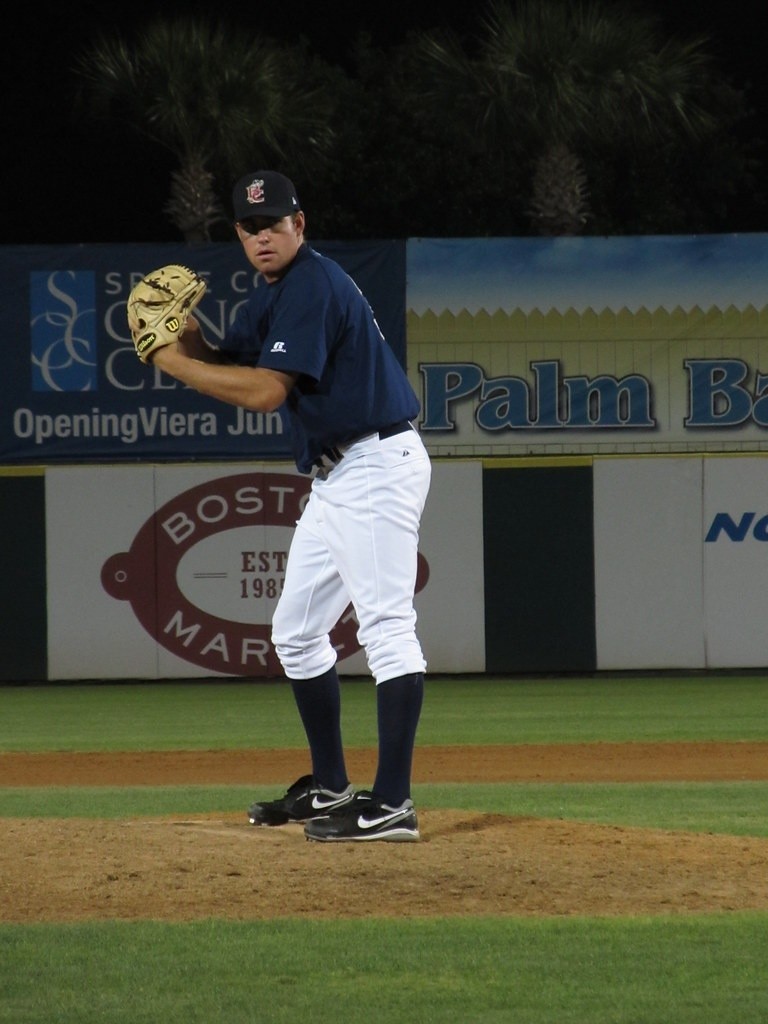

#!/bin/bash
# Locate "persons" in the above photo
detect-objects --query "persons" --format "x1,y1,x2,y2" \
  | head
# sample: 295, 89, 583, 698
125, 171, 430, 843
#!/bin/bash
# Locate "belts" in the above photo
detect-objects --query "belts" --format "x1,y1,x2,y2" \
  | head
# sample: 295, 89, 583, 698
314, 421, 413, 468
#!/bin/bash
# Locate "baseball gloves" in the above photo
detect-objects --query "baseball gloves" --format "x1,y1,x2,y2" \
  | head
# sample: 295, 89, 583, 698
126, 263, 207, 367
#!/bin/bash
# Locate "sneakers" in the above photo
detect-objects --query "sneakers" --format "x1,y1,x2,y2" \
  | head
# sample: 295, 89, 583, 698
247, 775, 353, 827
303, 790, 421, 843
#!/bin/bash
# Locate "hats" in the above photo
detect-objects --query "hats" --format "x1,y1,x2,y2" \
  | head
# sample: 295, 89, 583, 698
232, 170, 299, 221
